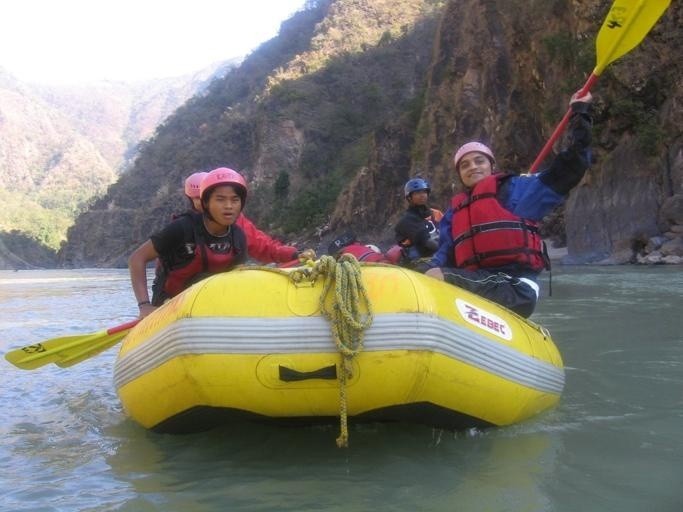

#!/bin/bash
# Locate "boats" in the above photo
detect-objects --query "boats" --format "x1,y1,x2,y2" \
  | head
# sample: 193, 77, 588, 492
113, 254, 565, 433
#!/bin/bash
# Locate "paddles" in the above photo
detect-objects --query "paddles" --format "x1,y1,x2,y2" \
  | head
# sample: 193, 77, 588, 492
6, 317, 140, 368
528, 1, 670, 175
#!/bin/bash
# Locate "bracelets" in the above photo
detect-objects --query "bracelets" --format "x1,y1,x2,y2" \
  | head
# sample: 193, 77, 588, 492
137, 301, 152, 306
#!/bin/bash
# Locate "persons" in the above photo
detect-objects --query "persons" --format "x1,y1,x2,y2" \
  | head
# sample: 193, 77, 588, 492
170, 170, 315, 264
128, 166, 317, 322
394, 179, 444, 268
412, 88, 595, 319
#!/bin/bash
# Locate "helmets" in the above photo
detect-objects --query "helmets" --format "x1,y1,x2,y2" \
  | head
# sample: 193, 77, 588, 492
198, 167, 248, 212
402, 178, 432, 202
453, 140, 496, 171
182, 171, 208, 199
